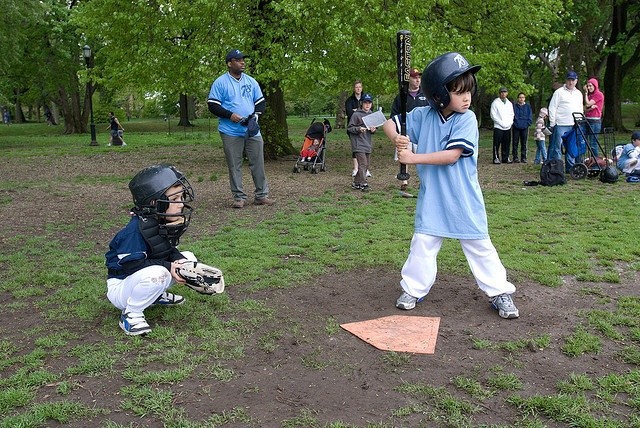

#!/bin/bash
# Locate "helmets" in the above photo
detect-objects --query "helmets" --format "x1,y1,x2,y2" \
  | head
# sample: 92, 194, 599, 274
129, 163, 194, 246
604, 167, 618, 182
539, 108, 548, 118
421, 52, 481, 112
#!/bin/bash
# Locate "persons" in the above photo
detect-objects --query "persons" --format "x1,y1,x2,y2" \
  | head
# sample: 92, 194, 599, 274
513, 92, 533, 163
390, 68, 430, 192
382, 50, 521, 316
206, 49, 275, 208
345, 81, 372, 178
346, 94, 377, 191
489, 88, 515, 164
616, 132, 640, 177
301, 137, 320, 162
106, 112, 127, 147
582, 78, 604, 158
105, 164, 198, 336
548, 72, 584, 174
533, 107, 549, 165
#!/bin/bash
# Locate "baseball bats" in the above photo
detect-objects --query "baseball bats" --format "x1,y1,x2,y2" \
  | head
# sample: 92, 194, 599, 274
397, 30, 411, 181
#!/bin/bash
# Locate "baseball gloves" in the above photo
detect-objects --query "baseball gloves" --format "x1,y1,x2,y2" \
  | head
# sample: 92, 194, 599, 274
174, 260, 225, 296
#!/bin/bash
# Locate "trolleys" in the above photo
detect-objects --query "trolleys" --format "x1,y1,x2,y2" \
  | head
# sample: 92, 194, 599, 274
569, 112, 617, 180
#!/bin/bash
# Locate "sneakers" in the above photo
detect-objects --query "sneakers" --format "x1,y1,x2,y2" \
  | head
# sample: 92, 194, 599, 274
254, 198, 276, 206
488, 293, 520, 318
534, 160, 539, 164
493, 158, 501, 164
119, 310, 152, 335
352, 170, 358, 177
396, 292, 424, 310
352, 182, 361, 189
505, 157, 512, 163
366, 170, 371, 177
401, 184, 407, 191
231, 199, 247, 208
521, 159, 528, 163
360, 183, 372, 192
513, 159, 520, 163
152, 292, 186, 305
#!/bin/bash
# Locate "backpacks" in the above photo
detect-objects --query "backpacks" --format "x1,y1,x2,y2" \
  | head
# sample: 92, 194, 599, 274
539, 159, 567, 187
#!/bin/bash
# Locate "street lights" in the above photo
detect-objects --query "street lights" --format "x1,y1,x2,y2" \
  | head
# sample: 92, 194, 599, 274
82, 45, 99, 145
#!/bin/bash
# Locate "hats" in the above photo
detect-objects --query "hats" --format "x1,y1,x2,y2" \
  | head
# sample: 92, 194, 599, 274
226, 50, 249, 61
410, 68, 422, 77
632, 132, 640, 138
567, 72, 578, 79
361, 94, 373, 102
499, 88, 507, 93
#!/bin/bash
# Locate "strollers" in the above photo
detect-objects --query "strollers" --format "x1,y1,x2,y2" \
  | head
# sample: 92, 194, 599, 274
292, 117, 333, 173
